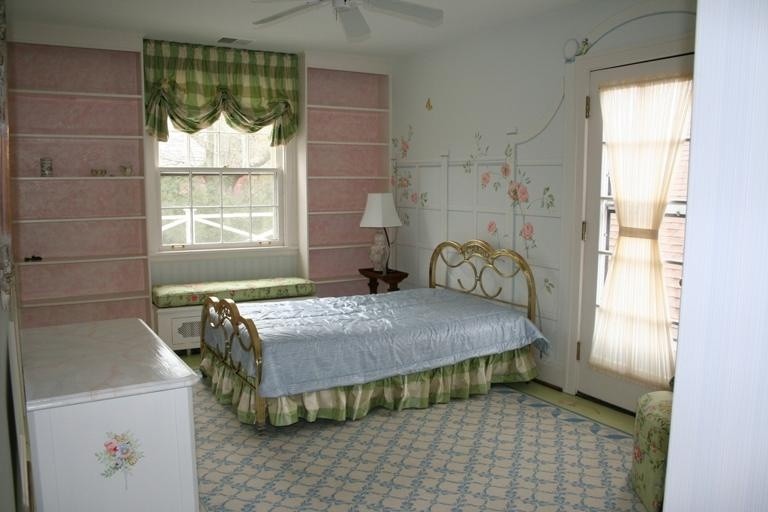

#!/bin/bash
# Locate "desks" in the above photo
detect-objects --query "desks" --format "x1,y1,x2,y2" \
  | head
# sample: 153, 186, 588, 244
19, 317, 199, 512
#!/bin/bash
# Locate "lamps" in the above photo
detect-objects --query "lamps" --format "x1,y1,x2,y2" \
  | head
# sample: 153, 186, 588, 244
360, 193, 402, 272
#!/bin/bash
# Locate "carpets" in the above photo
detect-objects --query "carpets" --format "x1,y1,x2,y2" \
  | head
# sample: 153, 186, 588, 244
193, 368, 647, 512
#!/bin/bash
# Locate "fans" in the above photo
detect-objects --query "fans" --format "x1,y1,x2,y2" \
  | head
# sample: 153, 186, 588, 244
253, 0, 443, 36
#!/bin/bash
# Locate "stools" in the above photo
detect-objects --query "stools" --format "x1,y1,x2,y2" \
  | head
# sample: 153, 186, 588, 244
629, 391, 674, 512
359, 268, 409, 294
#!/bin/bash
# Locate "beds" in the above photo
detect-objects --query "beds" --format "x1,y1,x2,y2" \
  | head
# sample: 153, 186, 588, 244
200, 240, 551, 430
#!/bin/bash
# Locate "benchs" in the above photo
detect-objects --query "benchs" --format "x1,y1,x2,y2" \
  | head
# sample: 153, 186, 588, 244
152, 276, 319, 356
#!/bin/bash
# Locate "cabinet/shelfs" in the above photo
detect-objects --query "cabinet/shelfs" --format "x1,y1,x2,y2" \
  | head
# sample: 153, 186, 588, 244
299, 66, 393, 298
8, 39, 154, 332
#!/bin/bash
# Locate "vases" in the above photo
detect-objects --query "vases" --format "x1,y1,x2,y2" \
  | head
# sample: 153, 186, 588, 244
369, 234, 388, 272
